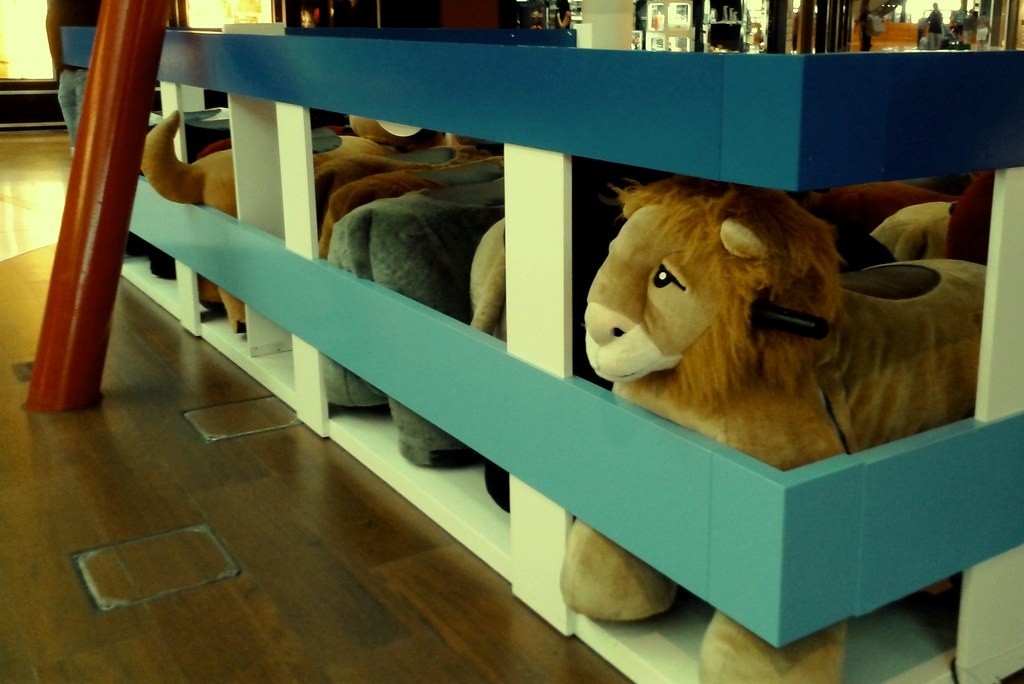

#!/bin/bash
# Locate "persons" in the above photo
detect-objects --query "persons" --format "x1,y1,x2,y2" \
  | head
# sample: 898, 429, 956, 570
854, 4, 978, 49
45, 0, 100, 157
530, 0, 572, 29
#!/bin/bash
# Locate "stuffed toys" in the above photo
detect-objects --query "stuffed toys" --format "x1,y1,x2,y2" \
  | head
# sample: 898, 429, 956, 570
141, 108, 994, 684
